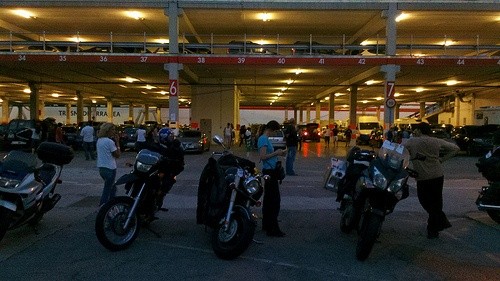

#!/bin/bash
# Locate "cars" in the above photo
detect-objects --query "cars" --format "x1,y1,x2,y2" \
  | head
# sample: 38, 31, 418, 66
0, 118, 206, 155
241, 115, 500, 153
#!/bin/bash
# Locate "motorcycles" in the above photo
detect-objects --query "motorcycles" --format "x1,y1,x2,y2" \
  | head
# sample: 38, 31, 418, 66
0, 141, 75, 243
204, 134, 271, 261
323, 139, 417, 262
94, 147, 186, 252
474, 147, 500, 225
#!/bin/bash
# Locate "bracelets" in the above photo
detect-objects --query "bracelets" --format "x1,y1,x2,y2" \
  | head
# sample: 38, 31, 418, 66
272, 152, 275, 157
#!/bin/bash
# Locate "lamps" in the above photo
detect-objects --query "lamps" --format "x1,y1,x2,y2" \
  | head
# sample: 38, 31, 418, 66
36, 142, 75, 164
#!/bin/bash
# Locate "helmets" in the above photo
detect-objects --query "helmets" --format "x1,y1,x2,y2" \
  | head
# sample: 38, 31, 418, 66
159, 127, 171, 140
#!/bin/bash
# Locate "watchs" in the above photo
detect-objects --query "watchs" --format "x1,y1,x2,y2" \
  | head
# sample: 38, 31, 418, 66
118, 147, 120, 149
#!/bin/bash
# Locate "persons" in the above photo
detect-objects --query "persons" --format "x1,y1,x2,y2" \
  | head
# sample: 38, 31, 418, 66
256, 120, 287, 236
222, 122, 235, 149
133, 127, 186, 224
333, 124, 339, 147
56, 120, 164, 160
396, 122, 460, 237
323, 125, 331, 149
239, 125, 251, 147
285, 118, 299, 176
483, 115, 488, 125
95, 123, 119, 212
370, 125, 412, 148
344, 126, 351, 148
29, 124, 42, 154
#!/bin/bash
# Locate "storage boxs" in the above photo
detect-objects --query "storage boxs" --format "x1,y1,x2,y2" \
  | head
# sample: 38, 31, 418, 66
323, 159, 344, 189
377, 141, 403, 168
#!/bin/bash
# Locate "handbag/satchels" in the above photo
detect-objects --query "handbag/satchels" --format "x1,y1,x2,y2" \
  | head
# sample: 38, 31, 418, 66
273, 161, 285, 184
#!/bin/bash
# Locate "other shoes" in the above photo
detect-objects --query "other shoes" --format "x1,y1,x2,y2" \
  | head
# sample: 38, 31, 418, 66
267, 231, 286, 237
